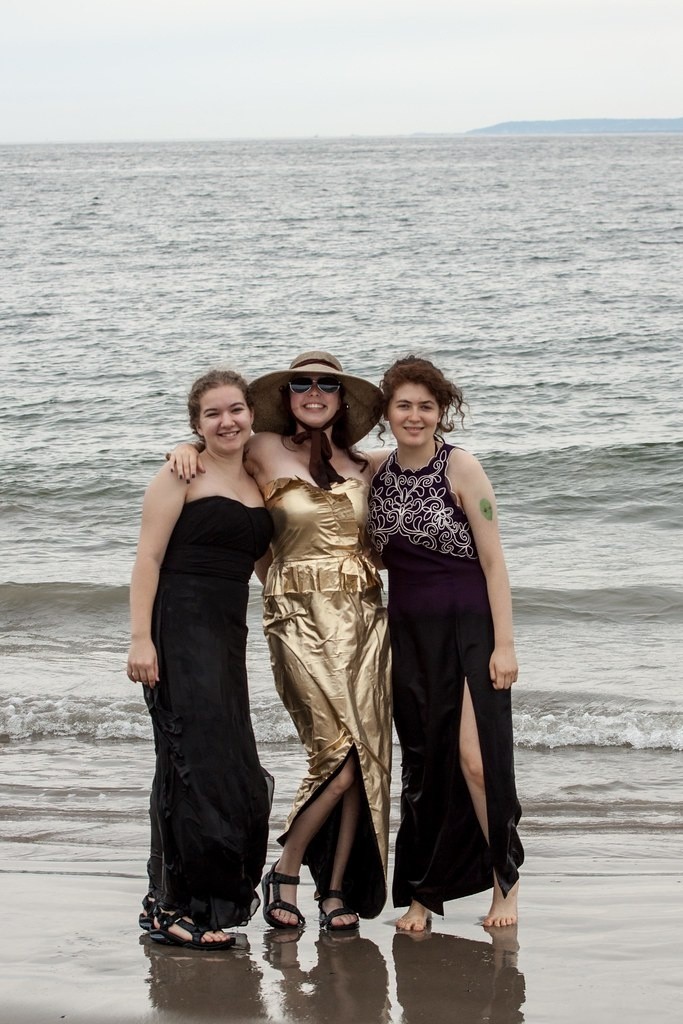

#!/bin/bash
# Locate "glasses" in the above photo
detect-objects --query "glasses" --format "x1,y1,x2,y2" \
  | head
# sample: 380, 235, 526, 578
285, 375, 342, 396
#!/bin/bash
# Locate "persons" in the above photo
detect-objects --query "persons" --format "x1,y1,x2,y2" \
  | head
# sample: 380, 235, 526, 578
363, 355, 526, 932
126, 368, 275, 949
166, 352, 398, 931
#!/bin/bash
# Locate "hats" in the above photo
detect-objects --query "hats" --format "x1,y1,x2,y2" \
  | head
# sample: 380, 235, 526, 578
245, 350, 386, 450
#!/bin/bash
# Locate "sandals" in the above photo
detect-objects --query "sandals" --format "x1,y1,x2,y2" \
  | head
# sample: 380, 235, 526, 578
317, 889, 361, 931
262, 928, 304, 971
261, 859, 306, 931
313, 932, 360, 955
138, 894, 237, 952
